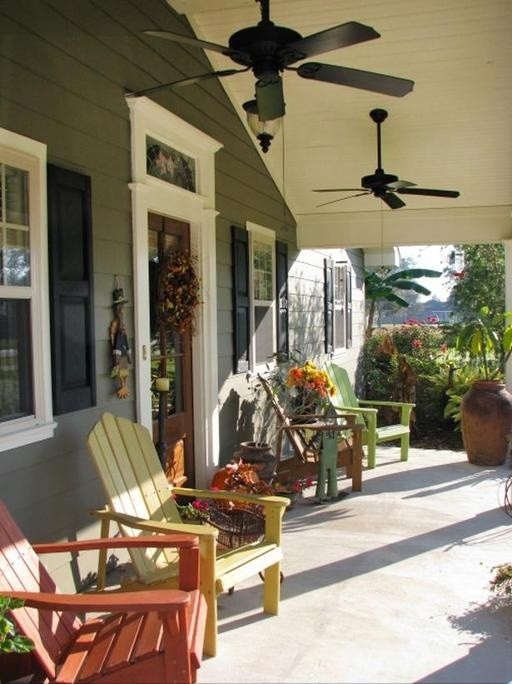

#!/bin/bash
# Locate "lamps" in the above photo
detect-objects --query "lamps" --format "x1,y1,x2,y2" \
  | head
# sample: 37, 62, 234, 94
241, 99, 285, 155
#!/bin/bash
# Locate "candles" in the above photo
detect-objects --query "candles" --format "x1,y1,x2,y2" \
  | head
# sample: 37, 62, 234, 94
155, 376, 170, 391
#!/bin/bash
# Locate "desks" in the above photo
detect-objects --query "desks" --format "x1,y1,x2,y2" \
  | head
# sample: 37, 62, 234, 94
197, 504, 284, 595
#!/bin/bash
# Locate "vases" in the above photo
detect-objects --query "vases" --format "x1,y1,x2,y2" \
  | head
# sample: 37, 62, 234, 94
233, 441, 279, 484
293, 389, 320, 425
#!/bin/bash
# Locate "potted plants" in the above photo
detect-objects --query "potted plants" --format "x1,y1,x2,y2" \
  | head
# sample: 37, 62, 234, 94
455, 306, 512, 466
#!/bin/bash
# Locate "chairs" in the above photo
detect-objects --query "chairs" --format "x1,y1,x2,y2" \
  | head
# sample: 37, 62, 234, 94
251, 371, 366, 492
0, 499, 209, 684
84, 411, 292, 657
320, 360, 416, 469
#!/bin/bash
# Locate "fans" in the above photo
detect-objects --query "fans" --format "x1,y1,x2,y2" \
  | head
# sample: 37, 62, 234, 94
124, 0, 415, 123
312, 108, 461, 209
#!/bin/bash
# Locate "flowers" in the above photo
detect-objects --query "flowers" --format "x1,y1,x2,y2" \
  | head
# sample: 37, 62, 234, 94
286, 359, 338, 399
240, 341, 331, 448
